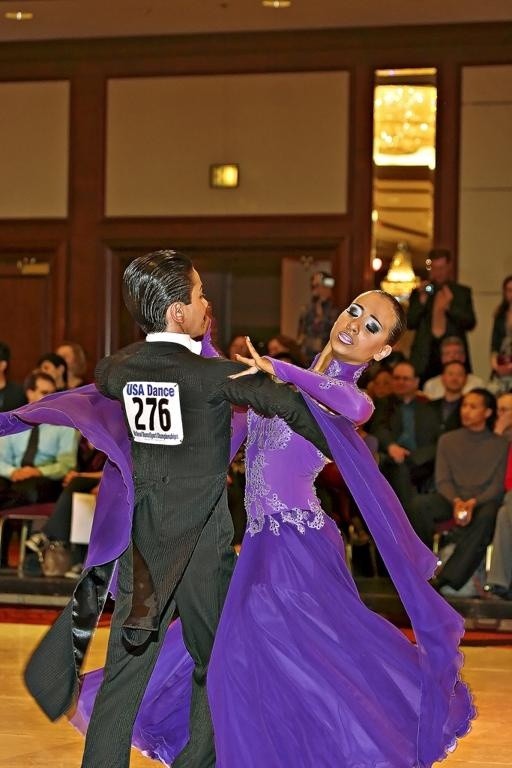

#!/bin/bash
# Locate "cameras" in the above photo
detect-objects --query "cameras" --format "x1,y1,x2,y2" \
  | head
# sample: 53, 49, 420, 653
425, 285, 434, 293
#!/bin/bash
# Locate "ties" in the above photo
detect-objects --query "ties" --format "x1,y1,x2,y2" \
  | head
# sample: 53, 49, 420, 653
21, 425, 41, 468
430, 285, 448, 339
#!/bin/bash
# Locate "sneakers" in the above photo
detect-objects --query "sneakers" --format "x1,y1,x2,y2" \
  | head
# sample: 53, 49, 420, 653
23, 532, 51, 553
63, 563, 85, 580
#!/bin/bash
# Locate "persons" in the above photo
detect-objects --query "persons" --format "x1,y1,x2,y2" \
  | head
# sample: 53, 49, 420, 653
221, 249, 510, 608
0, 285, 473, 767
79, 246, 339, 767
0, 340, 137, 578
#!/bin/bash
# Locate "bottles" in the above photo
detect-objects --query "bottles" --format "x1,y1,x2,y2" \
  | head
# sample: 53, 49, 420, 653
456, 504, 467, 522
